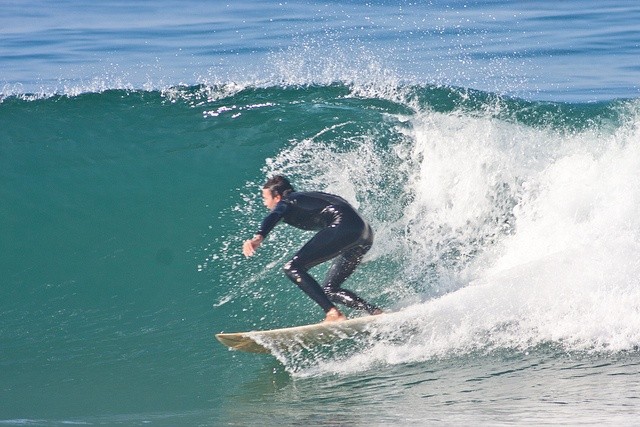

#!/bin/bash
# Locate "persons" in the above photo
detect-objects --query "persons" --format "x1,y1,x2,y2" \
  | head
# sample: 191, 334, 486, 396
242, 175, 383, 324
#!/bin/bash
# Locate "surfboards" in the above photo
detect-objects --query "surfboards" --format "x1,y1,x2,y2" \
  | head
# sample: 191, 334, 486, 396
214, 313, 388, 354
